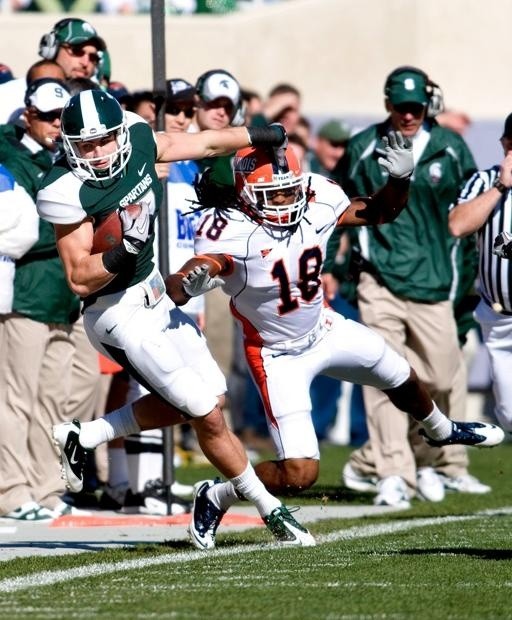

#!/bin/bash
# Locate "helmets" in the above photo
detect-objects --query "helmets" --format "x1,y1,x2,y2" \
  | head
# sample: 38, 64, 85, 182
234, 145, 308, 227
60, 89, 132, 182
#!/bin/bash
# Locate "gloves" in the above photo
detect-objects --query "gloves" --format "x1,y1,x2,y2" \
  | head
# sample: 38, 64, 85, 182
247, 122, 289, 168
182, 263, 226, 297
118, 202, 150, 254
376, 131, 414, 178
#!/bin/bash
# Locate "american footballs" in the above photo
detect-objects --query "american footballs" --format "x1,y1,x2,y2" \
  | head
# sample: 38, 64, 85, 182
91, 204, 142, 253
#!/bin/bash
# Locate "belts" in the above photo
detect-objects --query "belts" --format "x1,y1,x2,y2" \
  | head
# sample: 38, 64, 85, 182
479, 291, 512, 316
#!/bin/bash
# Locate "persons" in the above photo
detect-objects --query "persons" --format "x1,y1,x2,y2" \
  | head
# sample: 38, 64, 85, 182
162, 128, 507, 548
36, 88, 320, 551
0, 0, 512, 525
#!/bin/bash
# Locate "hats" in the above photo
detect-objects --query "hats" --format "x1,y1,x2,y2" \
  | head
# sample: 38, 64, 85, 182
319, 121, 349, 142
26, 18, 241, 112
385, 67, 430, 106
